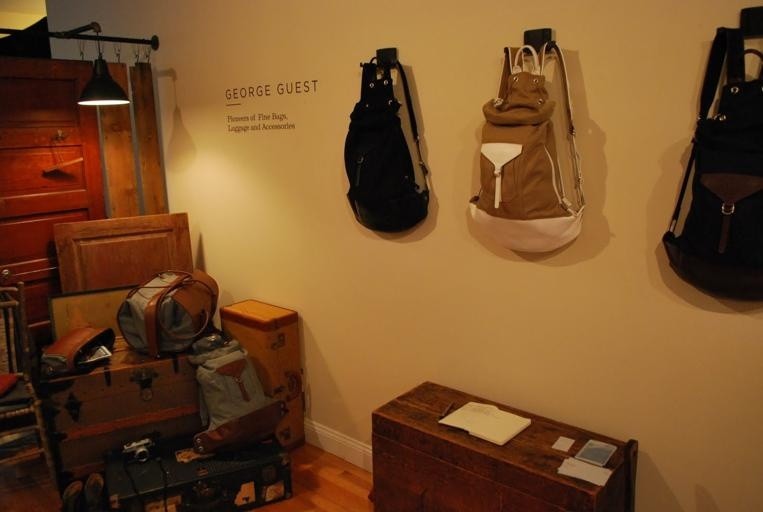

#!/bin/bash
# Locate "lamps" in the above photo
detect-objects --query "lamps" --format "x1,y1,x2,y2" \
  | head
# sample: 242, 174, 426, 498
76, 21, 131, 106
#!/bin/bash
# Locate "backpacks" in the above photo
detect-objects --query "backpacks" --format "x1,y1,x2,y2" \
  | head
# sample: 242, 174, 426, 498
468, 41, 585, 254
187, 334, 289, 454
116, 267, 218, 355
344, 58, 430, 231
663, 26, 763, 301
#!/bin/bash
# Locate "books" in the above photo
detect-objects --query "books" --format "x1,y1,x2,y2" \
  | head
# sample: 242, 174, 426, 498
437, 400, 533, 446
575, 438, 617, 466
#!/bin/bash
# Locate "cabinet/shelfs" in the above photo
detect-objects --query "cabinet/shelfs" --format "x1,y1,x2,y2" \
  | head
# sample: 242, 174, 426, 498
1, 55, 107, 341
371, 381, 640, 511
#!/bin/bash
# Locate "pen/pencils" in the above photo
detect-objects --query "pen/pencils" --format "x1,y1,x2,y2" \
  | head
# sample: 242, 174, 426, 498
438, 400, 454, 421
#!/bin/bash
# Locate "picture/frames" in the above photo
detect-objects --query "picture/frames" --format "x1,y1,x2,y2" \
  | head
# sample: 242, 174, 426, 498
46, 284, 138, 343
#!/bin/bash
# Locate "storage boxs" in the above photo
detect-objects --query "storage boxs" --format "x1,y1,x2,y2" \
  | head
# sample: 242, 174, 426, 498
218, 297, 306, 453
45, 342, 207, 466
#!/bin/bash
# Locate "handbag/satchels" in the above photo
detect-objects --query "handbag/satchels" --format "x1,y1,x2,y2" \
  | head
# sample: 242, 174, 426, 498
40, 322, 116, 378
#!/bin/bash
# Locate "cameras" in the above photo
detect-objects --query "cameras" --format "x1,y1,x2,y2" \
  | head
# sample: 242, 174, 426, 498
122, 438, 152, 462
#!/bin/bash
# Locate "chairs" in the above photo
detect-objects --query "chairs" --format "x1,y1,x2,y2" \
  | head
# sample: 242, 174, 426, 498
2, 281, 56, 479
